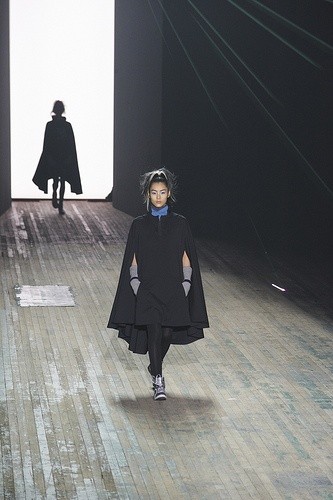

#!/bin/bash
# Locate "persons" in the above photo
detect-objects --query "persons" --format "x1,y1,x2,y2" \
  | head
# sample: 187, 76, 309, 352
33, 100, 82, 212
106, 170, 211, 401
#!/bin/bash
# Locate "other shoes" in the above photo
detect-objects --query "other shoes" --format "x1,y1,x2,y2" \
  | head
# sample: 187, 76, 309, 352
153, 374, 167, 400
